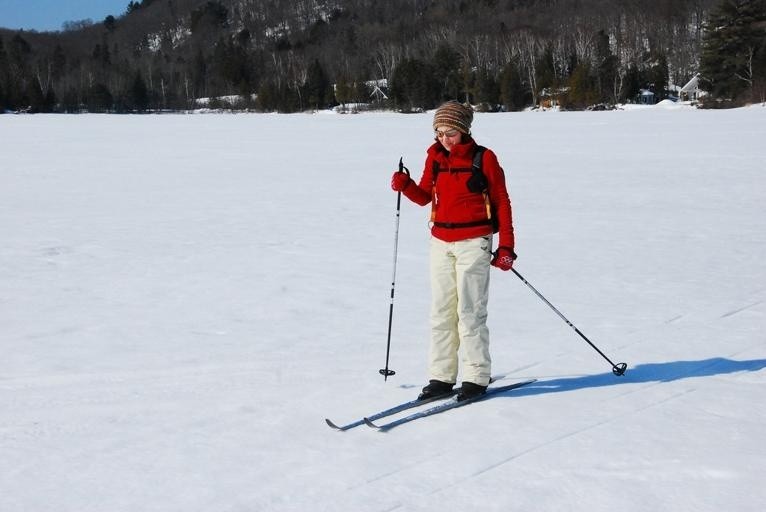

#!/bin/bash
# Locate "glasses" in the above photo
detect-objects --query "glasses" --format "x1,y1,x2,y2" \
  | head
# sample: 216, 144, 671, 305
435, 128, 458, 136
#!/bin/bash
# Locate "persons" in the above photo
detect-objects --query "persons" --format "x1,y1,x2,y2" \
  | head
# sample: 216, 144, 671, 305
391, 99, 516, 403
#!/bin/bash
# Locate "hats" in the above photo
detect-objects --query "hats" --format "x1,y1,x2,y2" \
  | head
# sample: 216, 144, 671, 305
433, 99, 473, 134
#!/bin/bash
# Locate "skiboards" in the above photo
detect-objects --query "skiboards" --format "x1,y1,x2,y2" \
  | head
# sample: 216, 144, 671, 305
326, 376, 537, 431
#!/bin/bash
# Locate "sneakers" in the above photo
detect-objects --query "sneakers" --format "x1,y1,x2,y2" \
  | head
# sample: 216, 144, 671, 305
422, 380, 454, 393
458, 382, 486, 396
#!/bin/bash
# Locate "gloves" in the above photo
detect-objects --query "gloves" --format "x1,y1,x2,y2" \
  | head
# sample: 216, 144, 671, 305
391, 172, 410, 191
489, 248, 517, 271
